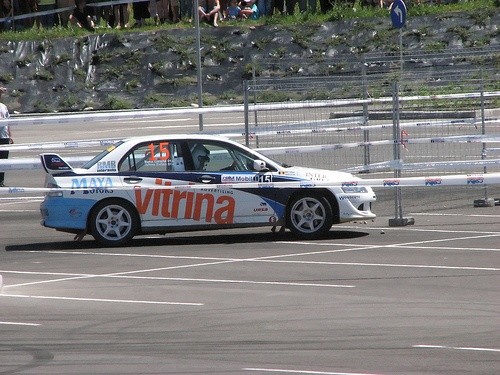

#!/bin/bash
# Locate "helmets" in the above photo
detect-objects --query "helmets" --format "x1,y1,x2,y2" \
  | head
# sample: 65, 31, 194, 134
192, 144, 210, 170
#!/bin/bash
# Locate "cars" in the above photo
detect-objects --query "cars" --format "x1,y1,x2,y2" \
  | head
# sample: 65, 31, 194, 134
40, 133, 378, 247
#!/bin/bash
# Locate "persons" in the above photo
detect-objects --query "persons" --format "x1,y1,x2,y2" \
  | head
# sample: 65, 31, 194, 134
0, 0, 459, 32
192, 144, 237, 171
0, 86, 14, 187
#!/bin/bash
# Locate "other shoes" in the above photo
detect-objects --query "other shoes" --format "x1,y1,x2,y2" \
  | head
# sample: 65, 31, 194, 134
0, 184, 5, 187
